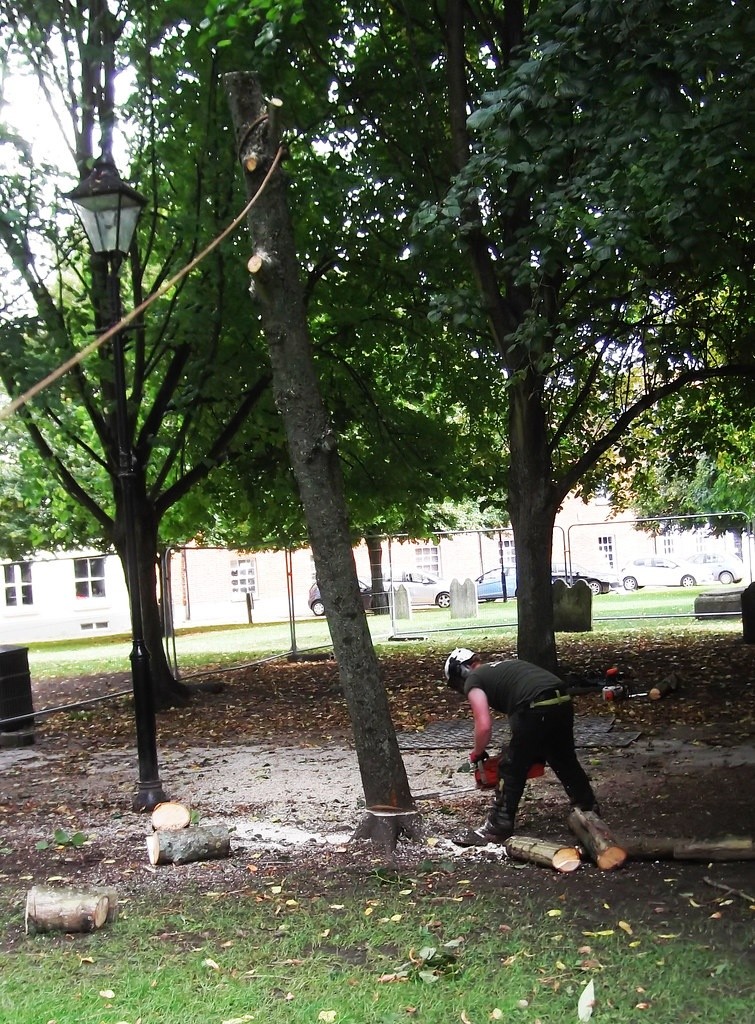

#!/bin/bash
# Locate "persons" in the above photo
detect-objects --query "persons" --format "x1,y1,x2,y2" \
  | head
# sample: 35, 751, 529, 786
445, 647, 602, 840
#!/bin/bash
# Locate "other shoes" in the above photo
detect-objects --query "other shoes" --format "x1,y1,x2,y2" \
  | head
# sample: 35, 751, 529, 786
477, 818, 514, 843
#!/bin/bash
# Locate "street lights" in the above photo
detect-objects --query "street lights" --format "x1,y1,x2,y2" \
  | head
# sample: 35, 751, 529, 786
61, 137, 170, 814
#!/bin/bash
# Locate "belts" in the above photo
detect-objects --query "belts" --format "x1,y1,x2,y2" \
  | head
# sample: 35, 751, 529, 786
525, 687, 574, 701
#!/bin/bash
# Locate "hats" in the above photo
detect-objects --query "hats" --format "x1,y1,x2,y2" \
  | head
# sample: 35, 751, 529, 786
440, 649, 480, 678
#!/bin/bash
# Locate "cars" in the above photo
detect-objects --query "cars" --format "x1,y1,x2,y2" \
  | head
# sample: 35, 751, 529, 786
307, 578, 390, 616
619, 556, 712, 592
552, 562, 618, 595
362, 569, 451, 609
681, 550, 744, 586
475, 566, 516, 602
